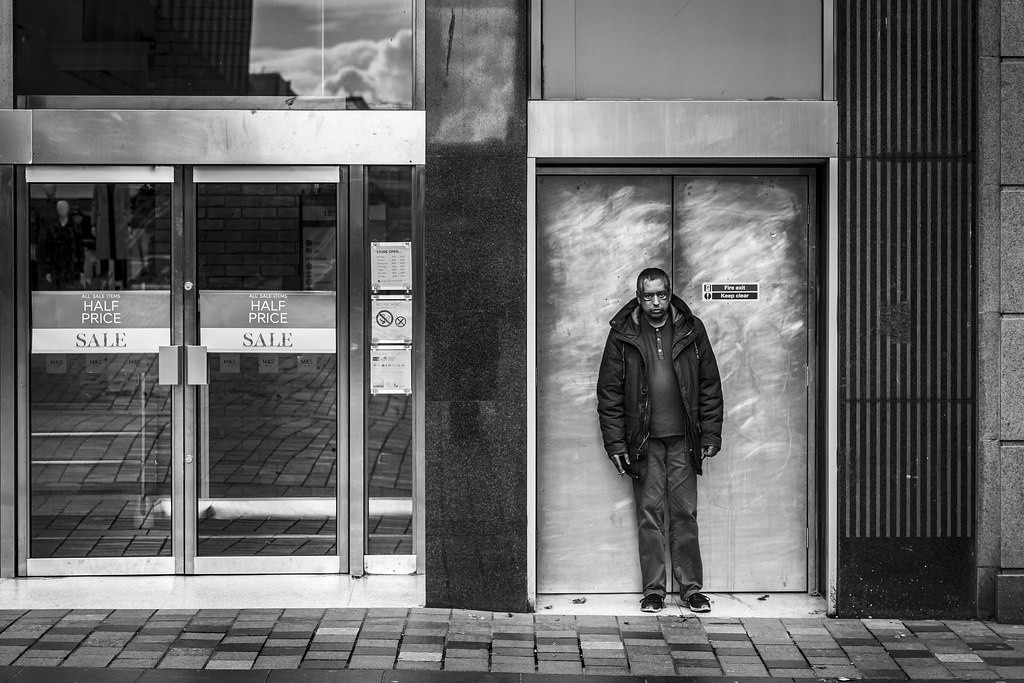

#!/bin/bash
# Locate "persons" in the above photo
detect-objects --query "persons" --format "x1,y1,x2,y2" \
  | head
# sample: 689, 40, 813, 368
596, 267, 725, 613
36, 197, 86, 332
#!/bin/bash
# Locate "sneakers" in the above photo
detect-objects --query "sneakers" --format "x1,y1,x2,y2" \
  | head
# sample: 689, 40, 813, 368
687, 593, 711, 612
641, 594, 666, 612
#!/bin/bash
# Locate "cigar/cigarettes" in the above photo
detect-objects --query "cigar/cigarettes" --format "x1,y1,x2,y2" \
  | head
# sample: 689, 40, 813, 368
617, 473, 621, 476
